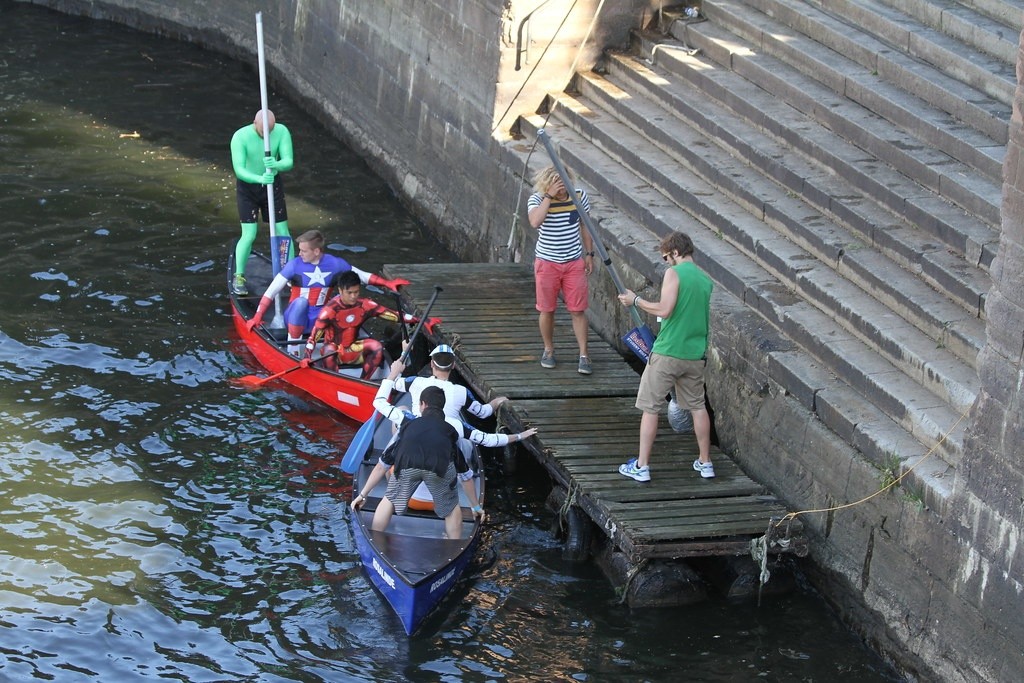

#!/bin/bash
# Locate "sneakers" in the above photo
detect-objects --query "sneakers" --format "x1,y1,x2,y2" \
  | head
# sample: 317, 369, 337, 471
232, 274, 249, 295
541, 350, 556, 368
578, 355, 593, 374
619, 457, 651, 481
693, 459, 715, 478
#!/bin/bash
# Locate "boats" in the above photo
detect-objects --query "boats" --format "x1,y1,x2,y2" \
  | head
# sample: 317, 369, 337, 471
226, 237, 396, 424
350, 363, 485, 637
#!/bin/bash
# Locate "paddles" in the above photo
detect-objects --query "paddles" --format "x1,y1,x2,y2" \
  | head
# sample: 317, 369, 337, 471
230, 345, 344, 389
339, 283, 443, 476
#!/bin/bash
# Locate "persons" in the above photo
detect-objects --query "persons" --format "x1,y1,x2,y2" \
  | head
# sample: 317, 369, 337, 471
230, 108, 297, 296
246, 231, 411, 356
526, 164, 596, 375
617, 231, 715, 481
300, 271, 442, 382
351, 344, 539, 540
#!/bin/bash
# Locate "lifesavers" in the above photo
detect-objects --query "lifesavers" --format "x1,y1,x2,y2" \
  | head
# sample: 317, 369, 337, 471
384, 330, 430, 377
486, 430, 521, 478
547, 507, 594, 565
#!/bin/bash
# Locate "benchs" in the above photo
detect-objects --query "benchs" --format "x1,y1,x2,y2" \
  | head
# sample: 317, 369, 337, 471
356, 495, 475, 523
260, 325, 314, 340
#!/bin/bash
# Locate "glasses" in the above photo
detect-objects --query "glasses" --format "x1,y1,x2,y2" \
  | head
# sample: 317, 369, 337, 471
662, 251, 671, 261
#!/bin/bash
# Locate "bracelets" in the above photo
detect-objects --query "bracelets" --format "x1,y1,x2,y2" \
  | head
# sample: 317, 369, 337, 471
544, 192, 554, 199
358, 492, 367, 501
471, 505, 481, 512
514, 433, 521, 442
633, 295, 641, 307
586, 251, 595, 257
